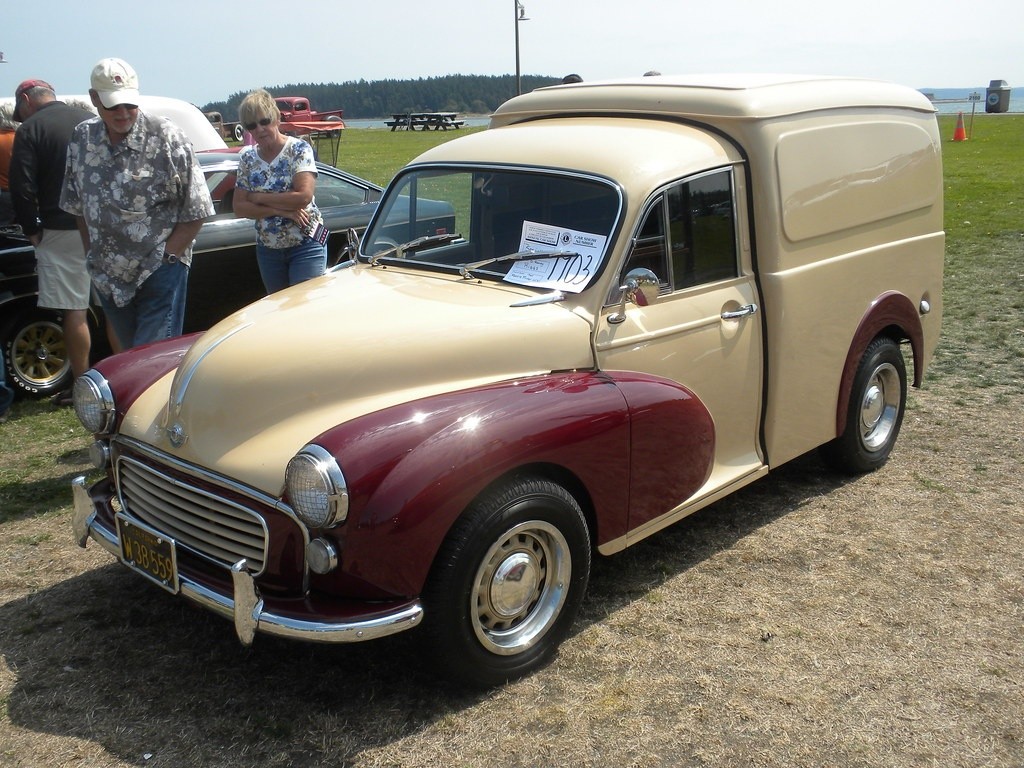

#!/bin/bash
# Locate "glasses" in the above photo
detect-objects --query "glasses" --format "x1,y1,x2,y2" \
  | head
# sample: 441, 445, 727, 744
102, 103, 137, 111
245, 119, 272, 130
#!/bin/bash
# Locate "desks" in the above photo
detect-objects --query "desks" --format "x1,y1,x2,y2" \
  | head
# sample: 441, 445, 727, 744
384, 113, 465, 131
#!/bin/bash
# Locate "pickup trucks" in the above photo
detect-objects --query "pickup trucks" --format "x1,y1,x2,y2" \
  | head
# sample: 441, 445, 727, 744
266, 97, 343, 141
202, 112, 245, 142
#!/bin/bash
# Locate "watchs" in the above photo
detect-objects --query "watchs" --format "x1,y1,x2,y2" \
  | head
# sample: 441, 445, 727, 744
163, 250, 177, 263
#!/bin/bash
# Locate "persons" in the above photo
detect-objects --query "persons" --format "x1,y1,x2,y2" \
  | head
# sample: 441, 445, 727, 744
58, 57, 217, 352
0, 101, 29, 221
8, 79, 127, 406
232, 88, 327, 295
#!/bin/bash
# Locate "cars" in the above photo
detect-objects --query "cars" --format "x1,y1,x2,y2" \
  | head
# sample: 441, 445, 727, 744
0, 151, 466, 401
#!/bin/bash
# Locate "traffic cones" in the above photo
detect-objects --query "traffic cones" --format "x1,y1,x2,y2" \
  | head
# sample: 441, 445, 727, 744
949, 112, 969, 142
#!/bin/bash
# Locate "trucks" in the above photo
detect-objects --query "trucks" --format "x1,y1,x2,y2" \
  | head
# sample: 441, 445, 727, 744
70, 76, 946, 687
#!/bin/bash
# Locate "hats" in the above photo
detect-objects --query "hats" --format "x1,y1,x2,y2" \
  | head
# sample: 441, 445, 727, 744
12, 80, 55, 122
92, 56, 140, 109
